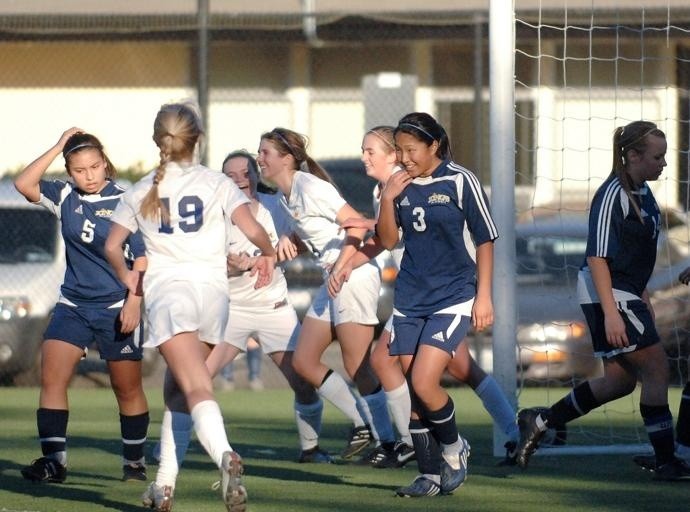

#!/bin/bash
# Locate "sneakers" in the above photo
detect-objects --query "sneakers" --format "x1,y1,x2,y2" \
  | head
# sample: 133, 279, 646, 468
21, 457, 65, 483
221, 378, 264, 392
395, 475, 441, 497
494, 408, 548, 470
299, 446, 336, 464
121, 443, 174, 512
631, 455, 690, 482
341, 424, 415, 468
211, 451, 248, 512
440, 432, 471, 493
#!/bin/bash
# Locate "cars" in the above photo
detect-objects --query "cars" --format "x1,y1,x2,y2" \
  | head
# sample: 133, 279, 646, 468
0, 172, 135, 385
259, 158, 396, 338
462, 206, 689, 385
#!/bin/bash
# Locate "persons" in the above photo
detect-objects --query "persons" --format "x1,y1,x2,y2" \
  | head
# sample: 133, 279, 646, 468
227, 127, 391, 468
219, 337, 265, 392
13, 125, 149, 483
516, 120, 690, 482
633, 263, 690, 472
103, 102, 276, 512
150, 150, 332, 466
336, 125, 521, 467
377, 112, 499, 497
